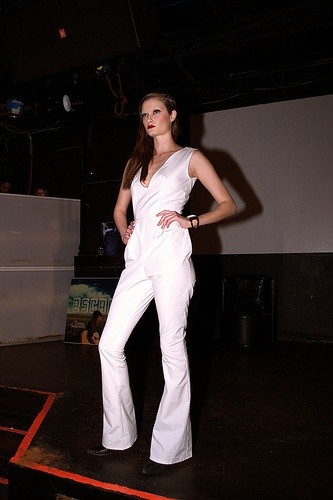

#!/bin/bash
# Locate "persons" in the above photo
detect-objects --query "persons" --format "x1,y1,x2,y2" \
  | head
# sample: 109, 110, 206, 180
86, 310, 102, 344
0, 178, 12, 192
88, 93, 235, 487
35, 187, 48, 196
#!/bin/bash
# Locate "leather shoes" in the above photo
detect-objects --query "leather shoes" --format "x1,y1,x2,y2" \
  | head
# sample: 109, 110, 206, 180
143, 459, 172, 476
88, 439, 139, 456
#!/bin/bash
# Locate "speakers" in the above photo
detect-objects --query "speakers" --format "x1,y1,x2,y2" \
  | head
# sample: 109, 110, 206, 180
223, 273, 278, 350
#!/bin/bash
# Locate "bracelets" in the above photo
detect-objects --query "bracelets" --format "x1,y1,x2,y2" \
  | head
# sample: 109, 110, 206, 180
186, 215, 199, 229
